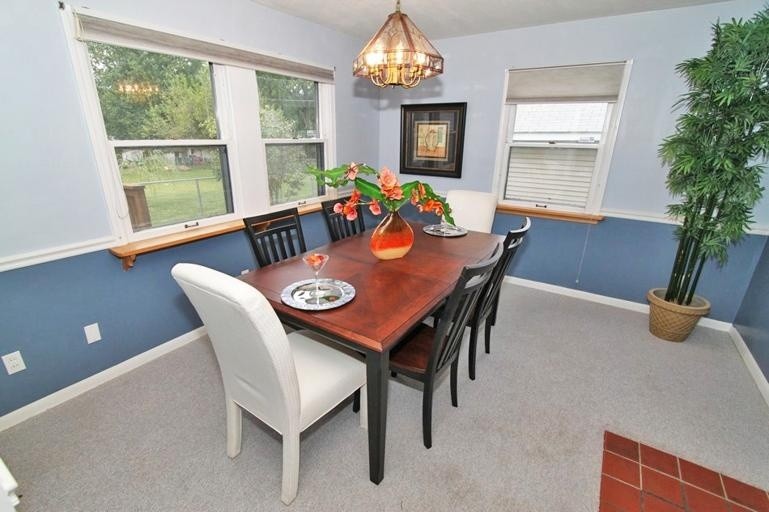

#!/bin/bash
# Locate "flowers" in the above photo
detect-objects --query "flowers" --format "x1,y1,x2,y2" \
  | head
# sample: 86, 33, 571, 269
303, 160, 456, 227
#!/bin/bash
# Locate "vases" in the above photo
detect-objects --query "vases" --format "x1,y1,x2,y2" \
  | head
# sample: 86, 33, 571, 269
370, 211, 415, 260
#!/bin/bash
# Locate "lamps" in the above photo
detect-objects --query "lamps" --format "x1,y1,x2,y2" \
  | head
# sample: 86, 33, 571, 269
352, 0, 444, 89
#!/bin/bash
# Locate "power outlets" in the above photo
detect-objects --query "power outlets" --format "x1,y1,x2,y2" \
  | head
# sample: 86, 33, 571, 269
1, 350, 26, 375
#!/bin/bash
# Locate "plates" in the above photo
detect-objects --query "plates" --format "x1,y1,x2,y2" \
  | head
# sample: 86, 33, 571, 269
422, 222, 468, 238
280, 276, 357, 312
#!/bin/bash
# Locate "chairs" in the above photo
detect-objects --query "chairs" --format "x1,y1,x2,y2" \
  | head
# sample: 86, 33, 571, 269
440, 189, 498, 233
353, 242, 504, 449
430, 217, 532, 380
321, 195, 366, 242
171, 263, 368, 507
243, 208, 307, 267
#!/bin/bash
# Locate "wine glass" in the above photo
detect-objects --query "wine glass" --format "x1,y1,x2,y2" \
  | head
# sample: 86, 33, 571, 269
302, 253, 330, 297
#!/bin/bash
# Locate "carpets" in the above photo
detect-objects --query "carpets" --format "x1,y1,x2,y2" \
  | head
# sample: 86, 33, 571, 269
599, 430, 769, 511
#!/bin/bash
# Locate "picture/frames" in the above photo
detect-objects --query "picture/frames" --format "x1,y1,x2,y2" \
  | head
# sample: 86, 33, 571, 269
399, 102, 467, 178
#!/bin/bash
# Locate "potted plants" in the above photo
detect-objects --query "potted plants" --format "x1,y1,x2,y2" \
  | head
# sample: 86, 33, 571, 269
646, 0, 769, 342
